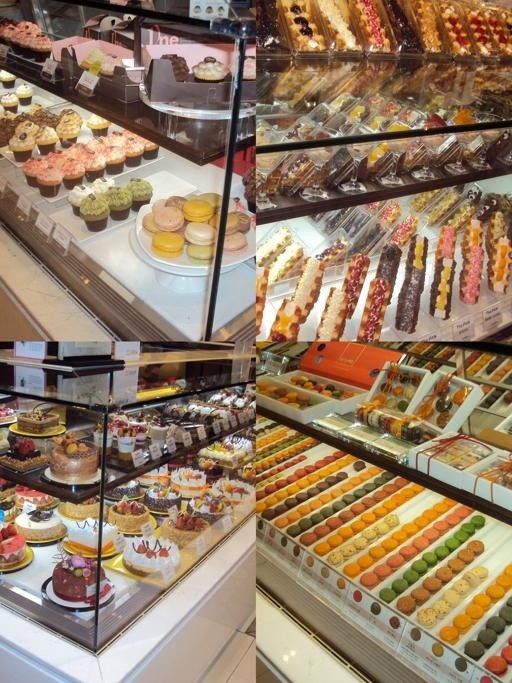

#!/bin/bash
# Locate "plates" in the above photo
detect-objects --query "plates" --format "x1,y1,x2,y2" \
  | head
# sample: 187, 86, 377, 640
6, 50, 45, 70
135, 195, 256, 271
47, 170, 198, 244
17, 148, 167, 202
1, 126, 92, 167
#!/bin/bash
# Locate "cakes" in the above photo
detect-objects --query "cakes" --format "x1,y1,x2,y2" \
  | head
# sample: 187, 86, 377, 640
192, 57, 230, 82
256, 1, 512, 342
0, 376, 256, 612
0, 70, 249, 264
0, 20, 53, 62
258, 343, 512, 683
230, 56, 257, 81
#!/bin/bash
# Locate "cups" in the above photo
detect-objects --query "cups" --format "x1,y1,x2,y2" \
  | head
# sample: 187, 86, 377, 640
11, 126, 110, 164
93, 416, 169, 463
0, 38, 52, 66
71, 199, 152, 231
24, 148, 161, 197
0, 79, 33, 114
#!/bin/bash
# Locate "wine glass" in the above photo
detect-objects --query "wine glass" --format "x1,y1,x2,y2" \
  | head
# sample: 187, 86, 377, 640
257, 113, 511, 209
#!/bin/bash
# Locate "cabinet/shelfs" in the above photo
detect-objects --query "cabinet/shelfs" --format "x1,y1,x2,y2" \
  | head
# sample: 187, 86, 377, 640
255, 343, 512, 683
257, 40, 511, 342
2, 344, 255, 683
0, 1, 256, 344
